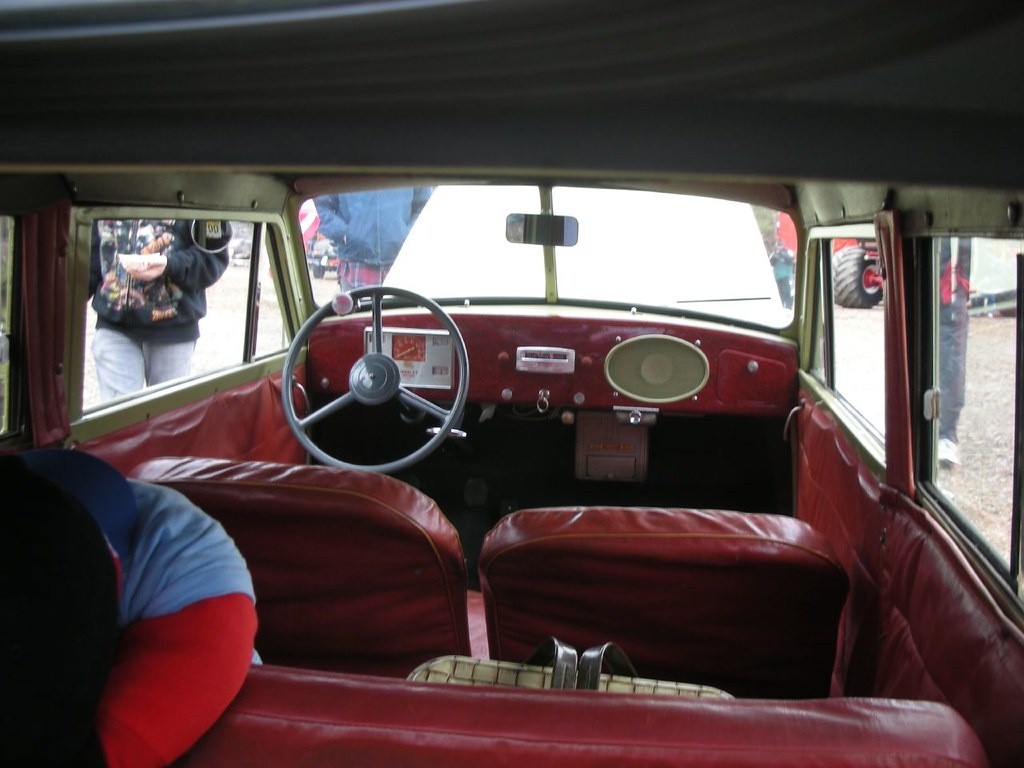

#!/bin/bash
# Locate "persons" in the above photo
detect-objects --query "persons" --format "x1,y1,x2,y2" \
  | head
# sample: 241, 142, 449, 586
1, 446, 262, 768
88, 217, 232, 401
938, 235, 972, 470
769, 240, 794, 311
313, 186, 435, 300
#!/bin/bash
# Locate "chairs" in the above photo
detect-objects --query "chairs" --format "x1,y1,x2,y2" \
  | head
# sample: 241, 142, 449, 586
124, 454, 466, 678
478, 507, 845, 700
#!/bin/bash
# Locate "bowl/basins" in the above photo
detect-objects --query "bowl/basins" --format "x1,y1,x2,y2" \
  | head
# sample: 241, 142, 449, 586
119, 252, 160, 274
